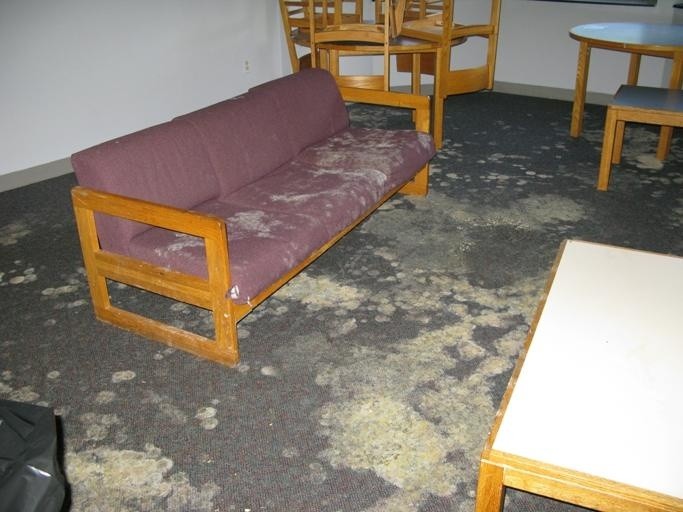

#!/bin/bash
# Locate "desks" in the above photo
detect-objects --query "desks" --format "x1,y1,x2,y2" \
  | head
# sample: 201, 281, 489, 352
285, 22, 468, 151
568, 22, 682, 161
474, 237, 683, 511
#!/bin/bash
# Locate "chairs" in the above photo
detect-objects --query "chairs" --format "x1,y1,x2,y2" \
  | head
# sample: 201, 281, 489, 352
279, 1, 501, 99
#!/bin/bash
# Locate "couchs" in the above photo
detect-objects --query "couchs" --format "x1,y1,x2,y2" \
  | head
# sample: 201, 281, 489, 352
67, 66, 430, 369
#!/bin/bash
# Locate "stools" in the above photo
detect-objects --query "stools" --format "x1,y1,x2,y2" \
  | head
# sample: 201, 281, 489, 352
596, 84, 683, 193
